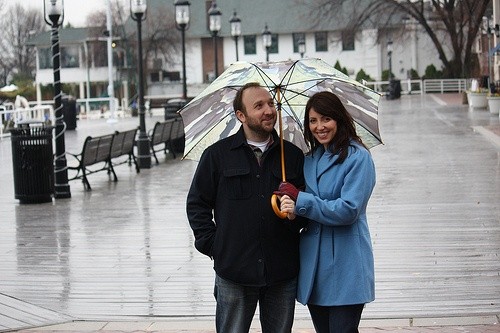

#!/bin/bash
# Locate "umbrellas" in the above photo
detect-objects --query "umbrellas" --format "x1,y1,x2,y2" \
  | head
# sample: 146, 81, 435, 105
177, 57, 385, 219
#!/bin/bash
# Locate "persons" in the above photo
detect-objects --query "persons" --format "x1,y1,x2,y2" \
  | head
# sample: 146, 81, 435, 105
272, 91, 376, 333
187, 83, 304, 333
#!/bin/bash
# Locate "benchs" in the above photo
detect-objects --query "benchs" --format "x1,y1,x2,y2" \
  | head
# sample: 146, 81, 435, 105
128, 118, 184, 166
53, 127, 141, 192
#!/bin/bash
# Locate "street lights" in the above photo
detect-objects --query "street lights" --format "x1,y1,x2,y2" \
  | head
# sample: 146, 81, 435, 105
229, 8, 243, 62
129, 0, 152, 169
261, 24, 272, 61
297, 36, 307, 58
42, 0, 72, 199
173, 0, 192, 99
385, 38, 394, 100
206, 3, 224, 79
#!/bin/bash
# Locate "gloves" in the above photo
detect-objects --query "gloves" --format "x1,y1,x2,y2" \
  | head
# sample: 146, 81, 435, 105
273, 181, 299, 202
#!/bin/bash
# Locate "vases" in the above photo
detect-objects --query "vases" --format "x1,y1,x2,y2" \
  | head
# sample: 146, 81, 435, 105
486, 96, 500, 114
467, 92, 488, 109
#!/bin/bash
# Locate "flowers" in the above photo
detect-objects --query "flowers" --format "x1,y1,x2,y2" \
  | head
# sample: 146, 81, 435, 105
486, 92, 500, 97
466, 86, 488, 93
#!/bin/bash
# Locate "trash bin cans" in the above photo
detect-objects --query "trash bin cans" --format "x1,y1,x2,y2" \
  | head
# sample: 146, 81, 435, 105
10, 122, 55, 204
390, 79, 400, 99
62, 95, 77, 131
